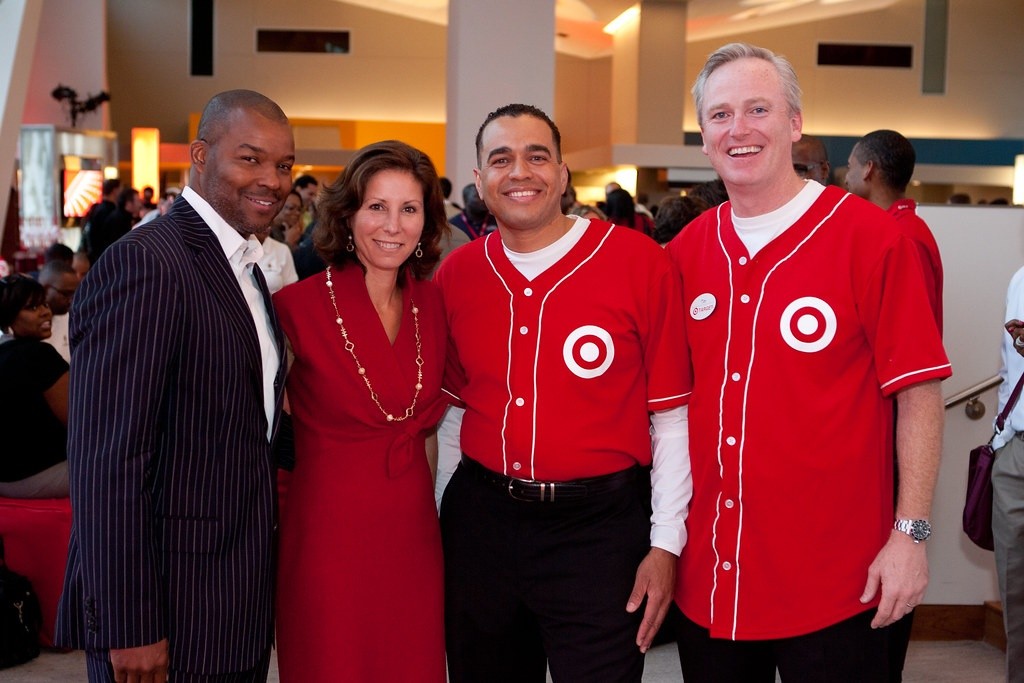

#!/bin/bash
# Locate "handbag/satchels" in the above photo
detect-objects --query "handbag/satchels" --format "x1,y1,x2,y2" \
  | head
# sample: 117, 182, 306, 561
962, 444, 996, 551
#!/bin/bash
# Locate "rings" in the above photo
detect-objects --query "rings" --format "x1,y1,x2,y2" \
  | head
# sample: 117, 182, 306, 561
907, 604, 916, 608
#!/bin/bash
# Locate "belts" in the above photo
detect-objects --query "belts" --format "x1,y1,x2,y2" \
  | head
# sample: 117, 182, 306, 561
460, 454, 639, 504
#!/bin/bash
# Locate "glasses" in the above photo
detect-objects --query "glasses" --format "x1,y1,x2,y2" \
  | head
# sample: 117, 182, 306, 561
50, 285, 75, 296
793, 162, 822, 175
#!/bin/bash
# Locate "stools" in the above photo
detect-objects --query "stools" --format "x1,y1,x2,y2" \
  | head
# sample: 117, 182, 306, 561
0, 496, 74, 650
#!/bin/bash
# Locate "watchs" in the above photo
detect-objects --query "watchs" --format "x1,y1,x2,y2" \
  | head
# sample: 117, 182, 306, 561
894, 520, 931, 543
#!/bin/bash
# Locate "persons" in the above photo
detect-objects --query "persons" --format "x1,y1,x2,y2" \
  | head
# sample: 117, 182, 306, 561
429, 177, 497, 280
843, 131, 943, 345
271, 174, 327, 278
30, 242, 89, 365
991, 262, 1024, 683
433, 103, 694, 683
0, 273, 70, 498
662, 44, 951, 683
562, 169, 729, 248
275, 141, 465, 683
792, 134, 830, 185
78, 180, 175, 266
56, 89, 295, 683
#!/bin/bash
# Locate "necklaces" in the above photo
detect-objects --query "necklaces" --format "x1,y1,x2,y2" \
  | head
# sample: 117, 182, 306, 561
326, 267, 425, 421
